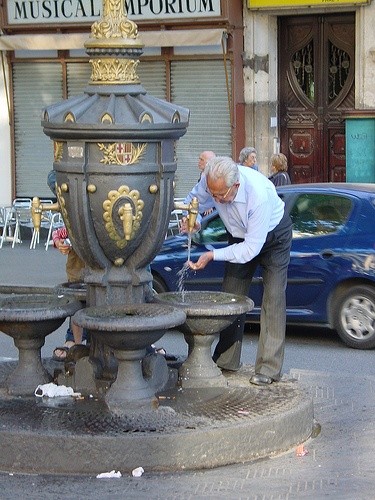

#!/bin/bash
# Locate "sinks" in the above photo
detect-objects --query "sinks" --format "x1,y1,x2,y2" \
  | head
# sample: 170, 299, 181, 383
0, 285, 82, 340
72, 301, 186, 352
155, 291, 254, 335
54, 280, 87, 301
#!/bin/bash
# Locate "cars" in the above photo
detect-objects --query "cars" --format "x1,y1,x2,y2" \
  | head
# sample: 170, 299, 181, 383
147, 182, 375, 350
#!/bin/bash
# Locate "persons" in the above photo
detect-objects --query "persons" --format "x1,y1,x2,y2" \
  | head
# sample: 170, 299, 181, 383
179, 155, 292, 387
196, 147, 292, 187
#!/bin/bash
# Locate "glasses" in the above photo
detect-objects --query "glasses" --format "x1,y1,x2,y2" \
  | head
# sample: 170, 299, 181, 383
205, 186, 233, 200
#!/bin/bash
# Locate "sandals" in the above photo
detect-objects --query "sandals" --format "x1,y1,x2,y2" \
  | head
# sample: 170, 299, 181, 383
151, 344, 166, 354
53, 340, 75, 361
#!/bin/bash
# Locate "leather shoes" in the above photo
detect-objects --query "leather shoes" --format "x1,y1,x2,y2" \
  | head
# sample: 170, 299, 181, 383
249, 373, 275, 386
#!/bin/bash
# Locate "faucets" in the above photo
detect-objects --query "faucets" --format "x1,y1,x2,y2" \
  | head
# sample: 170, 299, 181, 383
117, 203, 133, 240
174, 197, 199, 234
30, 196, 59, 231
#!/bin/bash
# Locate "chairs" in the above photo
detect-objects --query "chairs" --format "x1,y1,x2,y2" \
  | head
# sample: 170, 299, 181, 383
164, 197, 189, 240
0, 198, 72, 251
313, 204, 343, 234
